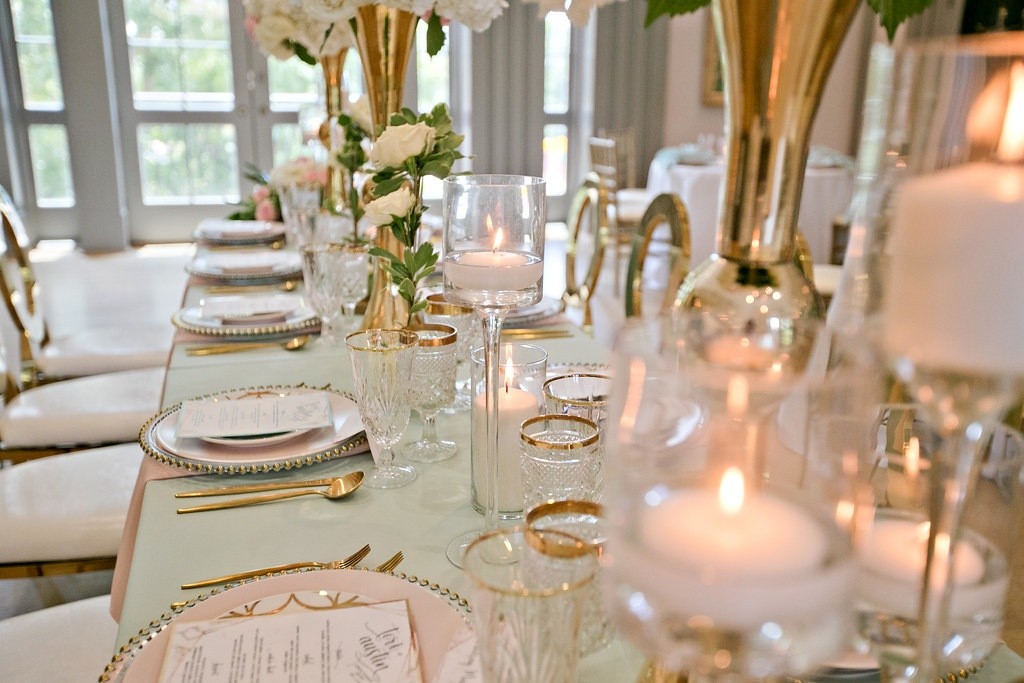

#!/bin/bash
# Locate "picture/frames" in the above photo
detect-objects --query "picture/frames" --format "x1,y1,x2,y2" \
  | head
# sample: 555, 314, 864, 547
697, 10, 724, 109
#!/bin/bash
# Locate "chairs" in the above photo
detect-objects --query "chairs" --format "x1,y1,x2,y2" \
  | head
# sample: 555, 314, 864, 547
813, 260, 843, 300
624, 190, 693, 318
586, 135, 622, 240
560, 169, 612, 338
597, 125, 651, 217
0, 181, 193, 683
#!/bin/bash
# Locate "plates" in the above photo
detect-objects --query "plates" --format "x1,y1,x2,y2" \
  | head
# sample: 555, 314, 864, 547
504, 295, 566, 324
138, 385, 367, 475
200, 429, 313, 448
193, 219, 284, 243
171, 305, 321, 336
98, 567, 474, 683
185, 251, 303, 279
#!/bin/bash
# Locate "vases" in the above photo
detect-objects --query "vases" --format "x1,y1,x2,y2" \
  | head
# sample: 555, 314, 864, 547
669, 0, 865, 378
319, 48, 349, 213
347, 2, 426, 330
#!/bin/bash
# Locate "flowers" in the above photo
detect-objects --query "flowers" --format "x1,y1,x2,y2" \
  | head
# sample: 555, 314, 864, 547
299, 0, 509, 59
221, 152, 327, 221
362, 101, 476, 327
334, 93, 373, 248
243, 0, 355, 66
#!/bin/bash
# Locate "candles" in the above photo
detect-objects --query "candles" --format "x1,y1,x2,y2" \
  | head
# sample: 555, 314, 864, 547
443, 226, 543, 292
469, 343, 548, 520
880, 58, 1024, 378
850, 519, 984, 619
627, 369, 823, 621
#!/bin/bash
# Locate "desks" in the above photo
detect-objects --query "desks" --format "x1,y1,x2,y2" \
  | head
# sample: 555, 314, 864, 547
645, 142, 855, 272
108, 219, 1024, 683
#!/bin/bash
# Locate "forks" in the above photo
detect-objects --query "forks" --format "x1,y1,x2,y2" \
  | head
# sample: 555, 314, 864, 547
181, 544, 371, 589
170, 551, 404, 608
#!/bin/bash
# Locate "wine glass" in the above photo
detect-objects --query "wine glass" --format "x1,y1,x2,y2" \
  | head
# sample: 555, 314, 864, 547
442, 172, 545, 577
426, 294, 474, 413
403, 323, 457, 463
850, 25, 1024, 683
345, 329, 417, 490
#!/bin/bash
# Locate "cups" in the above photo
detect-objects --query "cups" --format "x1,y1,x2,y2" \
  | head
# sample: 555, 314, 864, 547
861, 402, 1024, 665
465, 528, 597, 683
465, 343, 551, 522
542, 373, 612, 473
518, 414, 600, 522
470, 343, 549, 520
525, 500, 616, 657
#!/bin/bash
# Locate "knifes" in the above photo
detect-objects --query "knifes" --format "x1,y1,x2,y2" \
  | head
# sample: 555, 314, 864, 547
175, 477, 343, 497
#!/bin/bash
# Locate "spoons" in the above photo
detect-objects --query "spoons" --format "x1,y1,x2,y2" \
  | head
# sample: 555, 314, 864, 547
177, 471, 364, 514
186, 335, 310, 357
